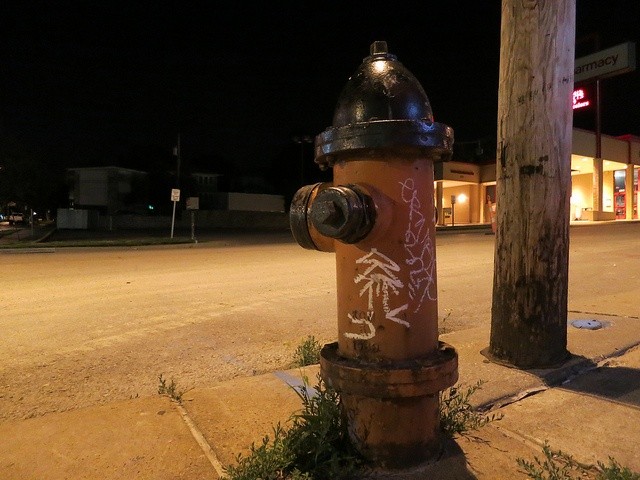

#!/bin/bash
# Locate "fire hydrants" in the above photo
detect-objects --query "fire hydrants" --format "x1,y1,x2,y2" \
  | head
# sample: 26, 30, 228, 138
288, 40, 466, 480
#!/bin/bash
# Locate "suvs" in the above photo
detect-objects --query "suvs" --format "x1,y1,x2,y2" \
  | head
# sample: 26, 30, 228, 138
9, 212, 25, 225
